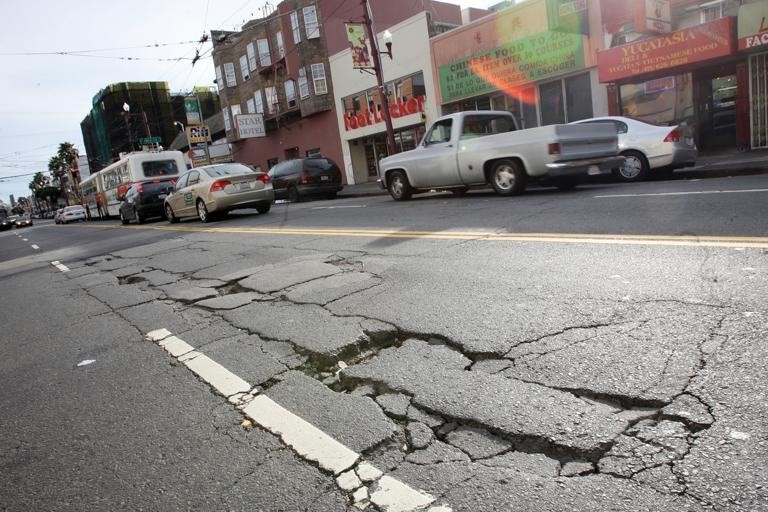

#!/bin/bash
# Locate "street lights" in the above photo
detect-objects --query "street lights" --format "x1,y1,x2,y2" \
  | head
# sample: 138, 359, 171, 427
344, 0, 398, 156
174, 121, 184, 132
122, 101, 134, 150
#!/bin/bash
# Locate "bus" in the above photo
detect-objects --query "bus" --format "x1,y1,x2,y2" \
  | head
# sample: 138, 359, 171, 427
79, 151, 187, 221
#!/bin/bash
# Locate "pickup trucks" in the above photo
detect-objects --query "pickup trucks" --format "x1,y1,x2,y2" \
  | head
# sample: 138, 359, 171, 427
377, 110, 626, 201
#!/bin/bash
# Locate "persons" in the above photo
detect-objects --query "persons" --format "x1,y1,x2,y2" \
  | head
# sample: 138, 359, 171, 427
83, 193, 91, 221
94, 192, 104, 221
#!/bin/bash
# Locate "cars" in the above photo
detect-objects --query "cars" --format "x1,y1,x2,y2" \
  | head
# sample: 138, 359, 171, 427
538, 116, 698, 187
0, 205, 88, 232
117, 158, 343, 224
